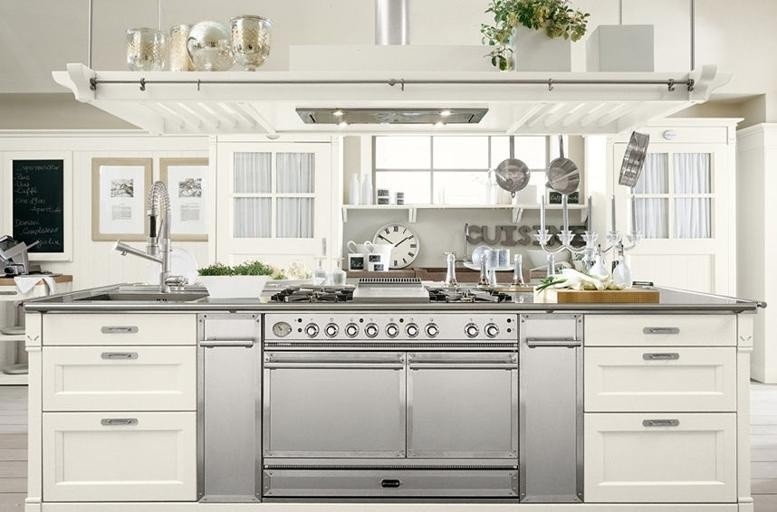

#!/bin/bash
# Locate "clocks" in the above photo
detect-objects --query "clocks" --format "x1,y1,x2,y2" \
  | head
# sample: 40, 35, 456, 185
372, 223, 420, 270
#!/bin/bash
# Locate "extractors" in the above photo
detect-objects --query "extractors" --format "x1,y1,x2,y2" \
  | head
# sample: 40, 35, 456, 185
295, 107, 489, 125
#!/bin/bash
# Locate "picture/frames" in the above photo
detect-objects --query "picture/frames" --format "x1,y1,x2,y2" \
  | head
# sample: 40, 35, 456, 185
91, 158, 150, 241
159, 158, 208, 241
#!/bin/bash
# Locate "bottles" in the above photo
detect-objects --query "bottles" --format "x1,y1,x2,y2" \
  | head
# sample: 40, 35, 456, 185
348, 173, 372, 206
590, 244, 634, 291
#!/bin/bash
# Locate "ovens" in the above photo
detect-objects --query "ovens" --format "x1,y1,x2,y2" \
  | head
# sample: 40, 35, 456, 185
261, 315, 523, 498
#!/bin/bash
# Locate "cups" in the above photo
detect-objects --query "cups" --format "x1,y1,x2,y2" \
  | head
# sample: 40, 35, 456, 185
485, 249, 512, 268
348, 254, 364, 274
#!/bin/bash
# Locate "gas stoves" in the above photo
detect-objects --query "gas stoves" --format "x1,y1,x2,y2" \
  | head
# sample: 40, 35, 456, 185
270, 285, 520, 304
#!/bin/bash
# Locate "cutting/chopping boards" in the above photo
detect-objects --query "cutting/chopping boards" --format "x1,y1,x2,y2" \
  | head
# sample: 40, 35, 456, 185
534, 285, 660, 306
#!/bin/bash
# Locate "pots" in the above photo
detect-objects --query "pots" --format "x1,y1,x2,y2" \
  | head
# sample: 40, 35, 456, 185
494, 137, 531, 198
544, 133, 580, 196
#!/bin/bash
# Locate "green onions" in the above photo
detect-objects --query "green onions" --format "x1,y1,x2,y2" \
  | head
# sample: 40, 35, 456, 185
536, 267, 627, 292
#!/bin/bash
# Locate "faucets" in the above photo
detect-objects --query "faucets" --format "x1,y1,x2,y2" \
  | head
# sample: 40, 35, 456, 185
148, 181, 171, 270
114, 241, 163, 265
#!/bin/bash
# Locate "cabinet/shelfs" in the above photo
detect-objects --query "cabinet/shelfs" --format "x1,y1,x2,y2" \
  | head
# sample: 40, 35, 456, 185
208, 133, 343, 279
24, 310, 197, 512
0, 274, 73, 386
583, 314, 753, 512
581, 117, 745, 299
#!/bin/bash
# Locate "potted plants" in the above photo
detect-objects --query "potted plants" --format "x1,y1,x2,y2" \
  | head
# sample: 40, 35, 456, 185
196, 260, 274, 298
479, 0, 590, 73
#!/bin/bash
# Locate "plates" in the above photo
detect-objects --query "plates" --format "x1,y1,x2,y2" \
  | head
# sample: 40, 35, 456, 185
462, 261, 520, 273
471, 246, 493, 267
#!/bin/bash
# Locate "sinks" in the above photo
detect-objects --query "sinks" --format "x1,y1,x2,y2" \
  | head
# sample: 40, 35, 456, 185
74, 293, 207, 302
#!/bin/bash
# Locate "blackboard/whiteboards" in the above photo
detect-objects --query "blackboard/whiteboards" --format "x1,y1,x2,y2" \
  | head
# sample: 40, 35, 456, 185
0, 149, 72, 262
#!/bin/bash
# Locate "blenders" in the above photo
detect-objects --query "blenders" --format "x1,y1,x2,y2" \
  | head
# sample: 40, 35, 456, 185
0, 234, 41, 278
